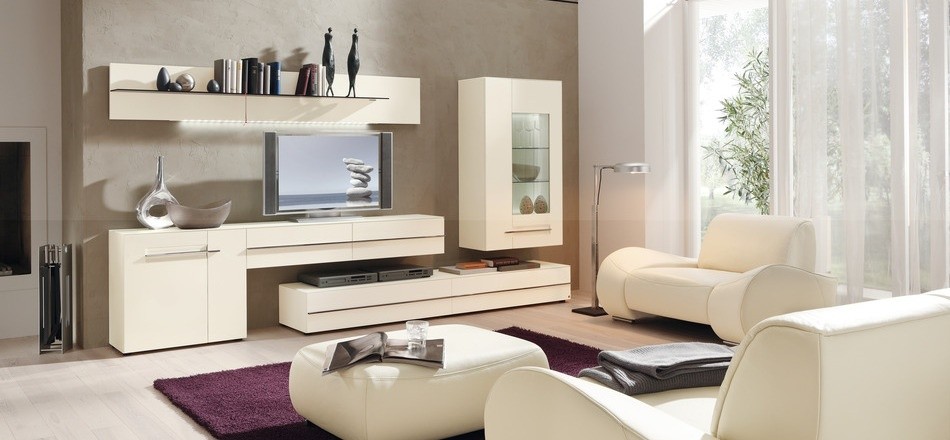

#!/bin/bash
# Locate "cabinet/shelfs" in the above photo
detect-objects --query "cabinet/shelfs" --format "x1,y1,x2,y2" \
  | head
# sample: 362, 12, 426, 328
108, 215, 570, 354
457, 77, 563, 251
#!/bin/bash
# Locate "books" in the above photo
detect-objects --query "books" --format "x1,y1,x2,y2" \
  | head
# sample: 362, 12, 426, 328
295, 63, 326, 97
439, 256, 540, 275
322, 331, 444, 375
214, 58, 281, 96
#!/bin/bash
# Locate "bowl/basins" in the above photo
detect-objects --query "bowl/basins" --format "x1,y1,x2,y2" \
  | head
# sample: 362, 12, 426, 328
164, 199, 232, 229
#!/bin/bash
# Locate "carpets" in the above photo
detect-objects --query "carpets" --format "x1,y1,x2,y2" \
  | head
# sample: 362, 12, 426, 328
153, 324, 603, 440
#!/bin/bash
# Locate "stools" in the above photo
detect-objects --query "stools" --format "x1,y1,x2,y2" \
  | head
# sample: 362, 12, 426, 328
287, 321, 549, 439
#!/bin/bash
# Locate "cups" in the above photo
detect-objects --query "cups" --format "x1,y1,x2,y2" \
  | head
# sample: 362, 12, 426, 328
406, 320, 430, 351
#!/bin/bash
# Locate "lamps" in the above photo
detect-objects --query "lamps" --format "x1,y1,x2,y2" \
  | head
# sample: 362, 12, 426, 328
571, 161, 651, 317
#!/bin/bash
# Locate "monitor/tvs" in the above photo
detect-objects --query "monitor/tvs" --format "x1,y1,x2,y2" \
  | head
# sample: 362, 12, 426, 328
261, 132, 394, 223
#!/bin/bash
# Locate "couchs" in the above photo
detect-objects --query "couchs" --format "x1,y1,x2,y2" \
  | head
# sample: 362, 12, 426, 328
483, 288, 950, 440
596, 214, 839, 343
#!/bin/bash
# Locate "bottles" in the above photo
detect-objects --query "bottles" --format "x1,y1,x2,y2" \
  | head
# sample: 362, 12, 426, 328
135, 155, 180, 230
